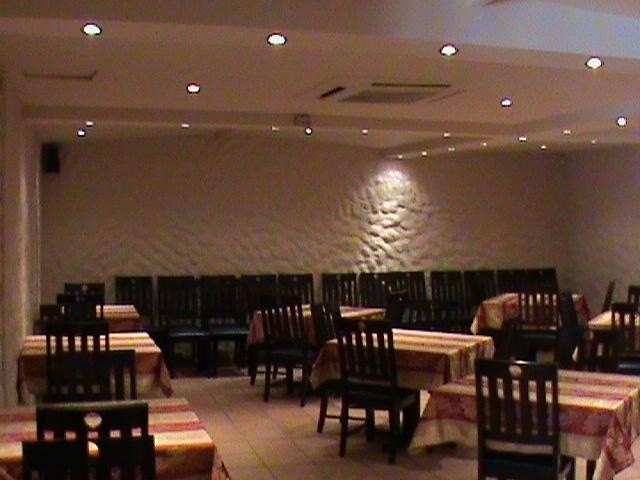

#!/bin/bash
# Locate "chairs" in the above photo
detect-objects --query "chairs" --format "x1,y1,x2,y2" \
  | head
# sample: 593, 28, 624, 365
560, 278, 640, 377
339, 318, 420, 467
114, 268, 560, 378
313, 303, 373, 431
473, 358, 577, 479
39, 283, 156, 479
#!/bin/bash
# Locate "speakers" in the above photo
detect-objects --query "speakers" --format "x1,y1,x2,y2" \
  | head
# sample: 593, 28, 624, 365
42, 145, 61, 174
